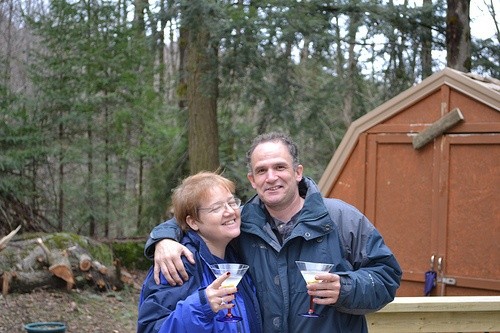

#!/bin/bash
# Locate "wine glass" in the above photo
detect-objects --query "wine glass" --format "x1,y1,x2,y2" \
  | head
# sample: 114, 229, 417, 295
295, 261, 335, 318
209, 263, 247, 322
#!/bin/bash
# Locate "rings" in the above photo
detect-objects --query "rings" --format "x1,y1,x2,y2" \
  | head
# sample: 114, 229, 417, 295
219, 297, 226, 306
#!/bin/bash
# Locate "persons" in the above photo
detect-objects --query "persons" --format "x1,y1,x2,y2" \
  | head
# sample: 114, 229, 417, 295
142, 131, 403, 333
136, 164, 265, 333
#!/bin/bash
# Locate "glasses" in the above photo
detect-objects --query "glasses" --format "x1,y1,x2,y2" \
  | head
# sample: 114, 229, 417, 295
197, 197, 243, 212
